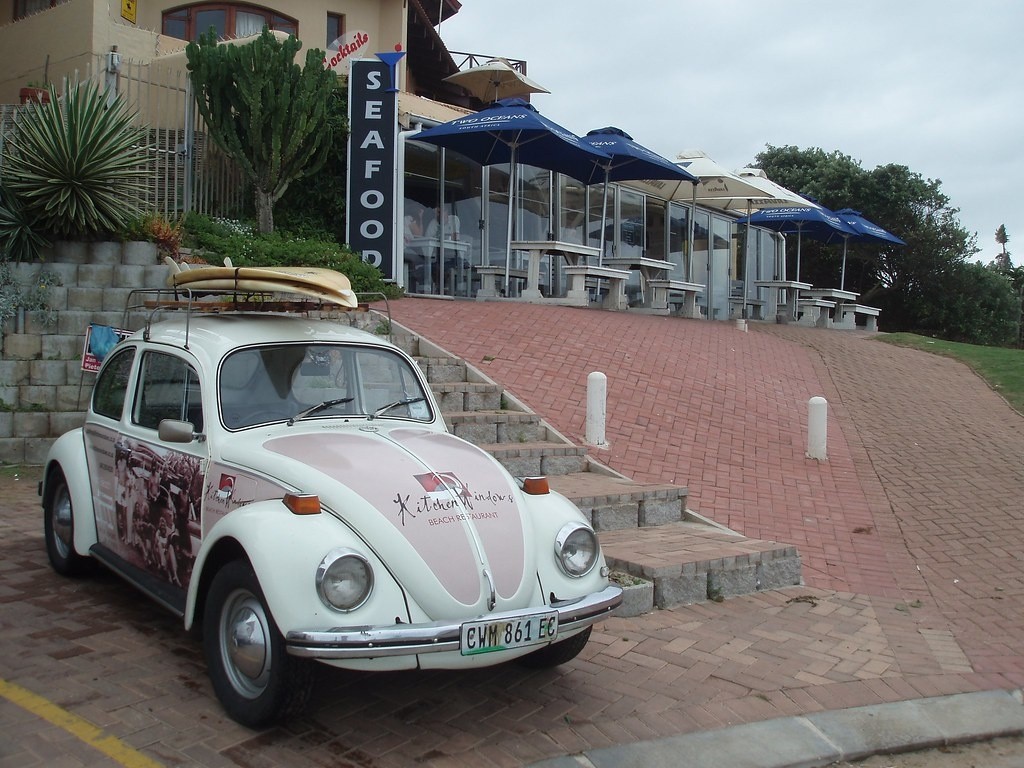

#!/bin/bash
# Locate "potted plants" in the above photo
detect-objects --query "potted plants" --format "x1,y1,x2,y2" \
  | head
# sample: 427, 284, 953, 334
19, 81, 60, 104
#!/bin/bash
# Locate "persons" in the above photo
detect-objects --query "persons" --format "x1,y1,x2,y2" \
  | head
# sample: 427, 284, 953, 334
116, 450, 204, 588
404, 204, 434, 295
425, 204, 460, 294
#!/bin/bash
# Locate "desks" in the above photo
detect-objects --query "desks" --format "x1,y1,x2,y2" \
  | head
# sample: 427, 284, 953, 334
476, 240, 861, 328
410, 236, 473, 297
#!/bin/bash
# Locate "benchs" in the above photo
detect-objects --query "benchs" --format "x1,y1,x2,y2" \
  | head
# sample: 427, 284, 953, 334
475, 265, 882, 331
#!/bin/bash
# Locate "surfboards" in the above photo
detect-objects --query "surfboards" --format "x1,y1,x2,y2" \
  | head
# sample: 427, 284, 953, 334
162, 256, 360, 310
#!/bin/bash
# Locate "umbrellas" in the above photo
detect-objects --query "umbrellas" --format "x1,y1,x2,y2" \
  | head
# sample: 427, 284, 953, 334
560, 125, 698, 300
733, 193, 861, 314
615, 148, 774, 283
682, 166, 819, 316
805, 206, 905, 290
407, 96, 614, 300
440, 56, 552, 105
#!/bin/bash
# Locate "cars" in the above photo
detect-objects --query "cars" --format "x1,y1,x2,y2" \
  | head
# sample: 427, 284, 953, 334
37, 286, 624, 734
